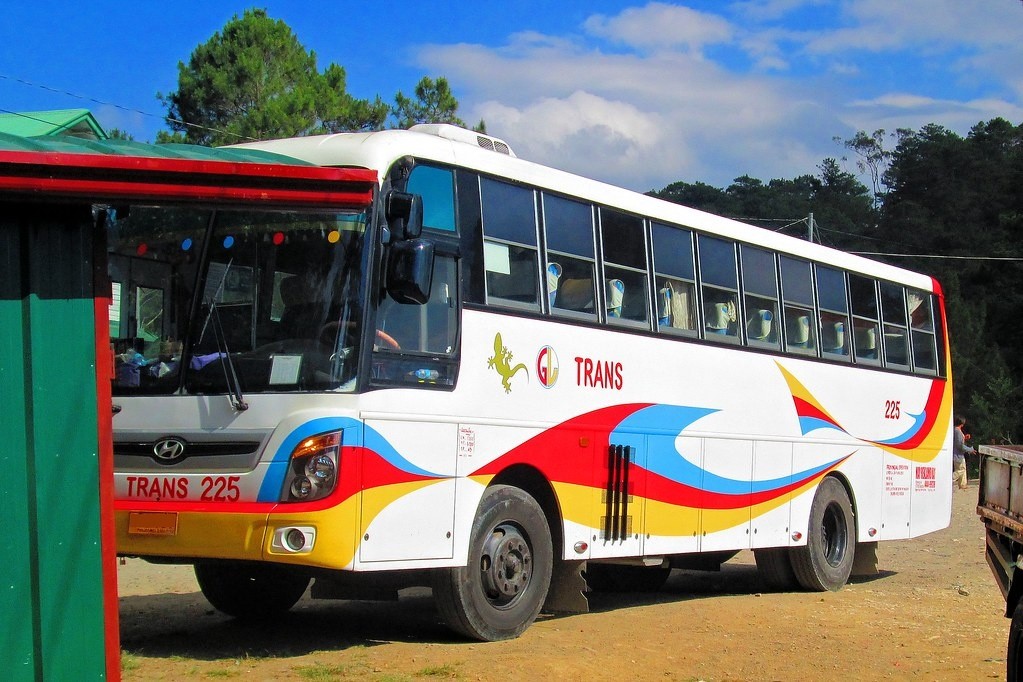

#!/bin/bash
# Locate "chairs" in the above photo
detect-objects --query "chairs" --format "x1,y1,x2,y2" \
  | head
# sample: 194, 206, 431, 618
823, 322, 844, 354
853, 327, 876, 359
557, 278, 625, 317
490, 258, 562, 306
786, 315, 809, 348
746, 309, 773, 341
621, 288, 672, 327
277, 276, 324, 338
884, 334, 905, 361
704, 302, 729, 335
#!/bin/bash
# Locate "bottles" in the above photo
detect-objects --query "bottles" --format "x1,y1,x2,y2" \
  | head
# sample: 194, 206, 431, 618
416, 369, 439, 380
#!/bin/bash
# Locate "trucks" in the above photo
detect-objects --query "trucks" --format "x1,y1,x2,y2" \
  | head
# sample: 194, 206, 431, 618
978, 444, 1023, 682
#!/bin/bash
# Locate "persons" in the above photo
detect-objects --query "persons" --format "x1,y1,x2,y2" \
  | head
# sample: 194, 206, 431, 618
953, 414, 976, 490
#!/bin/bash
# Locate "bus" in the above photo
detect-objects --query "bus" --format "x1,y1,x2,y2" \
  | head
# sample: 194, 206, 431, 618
112, 124, 956, 645
